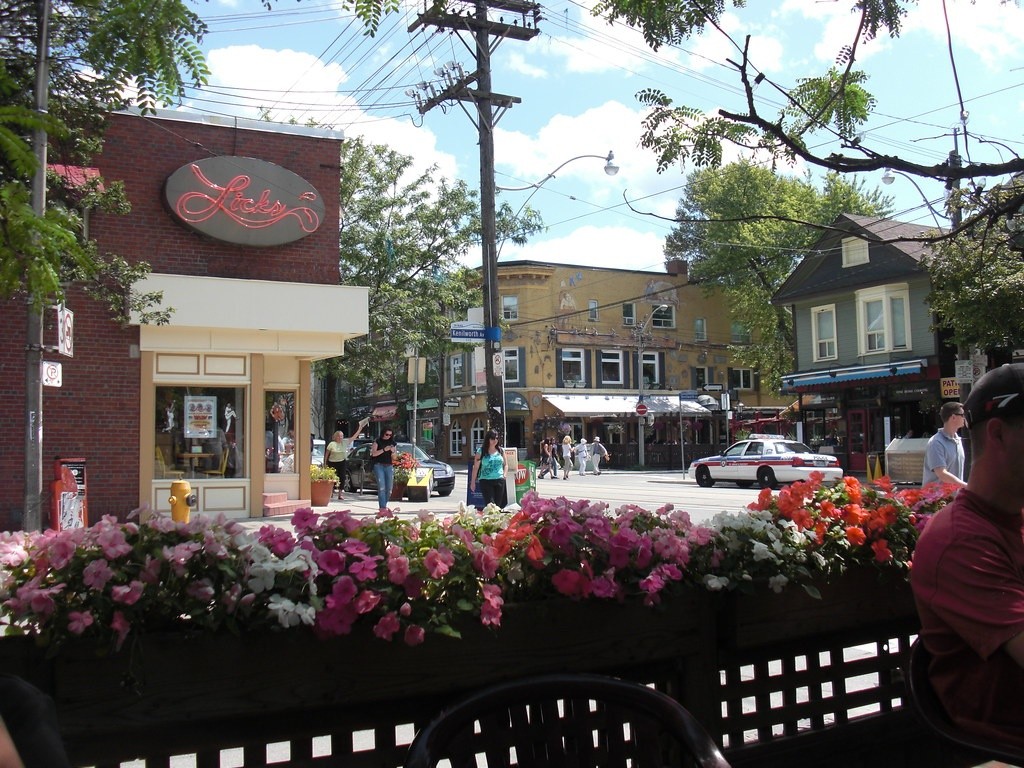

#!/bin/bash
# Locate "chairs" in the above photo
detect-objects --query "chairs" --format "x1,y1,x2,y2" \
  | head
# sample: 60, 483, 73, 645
201, 447, 229, 479
155, 447, 186, 480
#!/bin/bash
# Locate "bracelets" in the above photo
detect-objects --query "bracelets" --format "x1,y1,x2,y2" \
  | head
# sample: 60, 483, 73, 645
383, 448, 385, 452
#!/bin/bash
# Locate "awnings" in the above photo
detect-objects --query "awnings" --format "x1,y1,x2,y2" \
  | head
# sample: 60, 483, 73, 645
336, 404, 401, 424
782, 359, 928, 396
505, 392, 529, 411
542, 395, 721, 417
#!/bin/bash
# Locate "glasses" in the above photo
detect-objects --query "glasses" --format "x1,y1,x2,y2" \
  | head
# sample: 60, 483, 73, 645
386, 434, 392, 436
491, 438, 498, 440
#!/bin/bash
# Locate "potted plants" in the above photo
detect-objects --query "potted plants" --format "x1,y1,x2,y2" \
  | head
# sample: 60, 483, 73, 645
607, 424, 626, 434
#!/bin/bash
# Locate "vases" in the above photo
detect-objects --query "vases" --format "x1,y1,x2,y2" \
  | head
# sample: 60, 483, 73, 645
564, 431, 570, 434
311, 478, 335, 507
389, 481, 407, 502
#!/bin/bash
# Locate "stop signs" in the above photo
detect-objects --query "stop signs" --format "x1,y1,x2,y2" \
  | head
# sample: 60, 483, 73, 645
636, 404, 647, 416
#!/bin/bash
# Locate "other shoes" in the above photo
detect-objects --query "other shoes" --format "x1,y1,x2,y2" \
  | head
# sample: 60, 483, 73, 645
551, 476, 557, 479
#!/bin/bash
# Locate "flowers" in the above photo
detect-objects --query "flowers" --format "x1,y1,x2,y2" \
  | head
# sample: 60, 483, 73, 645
391, 451, 421, 482
677, 420, 692, 432
558, 423, 571, 431
309, 465, 342, 487
693, 421, 703, 429
0, 470, 967, 646
652, 421, 664, 429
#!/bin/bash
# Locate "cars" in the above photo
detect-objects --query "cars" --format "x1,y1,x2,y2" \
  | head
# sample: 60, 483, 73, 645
688, 439, 843, 489
343, 442, 455, 496
309, 439, 327, 466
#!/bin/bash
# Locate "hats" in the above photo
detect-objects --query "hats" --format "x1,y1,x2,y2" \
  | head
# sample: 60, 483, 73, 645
962, 363, 1024, 429
594, 437, 600, 442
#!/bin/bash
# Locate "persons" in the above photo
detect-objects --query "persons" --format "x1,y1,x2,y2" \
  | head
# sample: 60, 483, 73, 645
538, 436, 609, 480
921, 402, 968, 488
371, 425, 397, 511
324, 424, 362, 500
627, 438, 688, 456
910, 363, 1024, 768
0, 669, 69, 768
265, 421, 314, 473
198, 427, 228, 475
471, 428, 508, 508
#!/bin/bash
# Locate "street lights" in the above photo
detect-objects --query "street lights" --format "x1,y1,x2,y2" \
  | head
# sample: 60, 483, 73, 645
484, 154, 620, 456
638, 304, 669, 466
880, 165, 973, 482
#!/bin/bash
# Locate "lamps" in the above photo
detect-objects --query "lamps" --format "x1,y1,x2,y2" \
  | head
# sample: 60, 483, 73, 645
607, 327, 617, 338
568, 326, 578, 335
587, 327, 599, 336
829, 362, 844, 378
461, 433, 467, 447
889, 356, 906, 376
550, 324, 558, 335
785, 373, 794, 386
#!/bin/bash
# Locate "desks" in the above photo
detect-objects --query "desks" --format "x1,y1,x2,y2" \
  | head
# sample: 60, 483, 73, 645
176, 453, 214, 479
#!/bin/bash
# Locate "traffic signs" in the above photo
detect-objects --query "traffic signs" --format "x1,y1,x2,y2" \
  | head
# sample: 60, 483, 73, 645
702, 384, 723, 391
